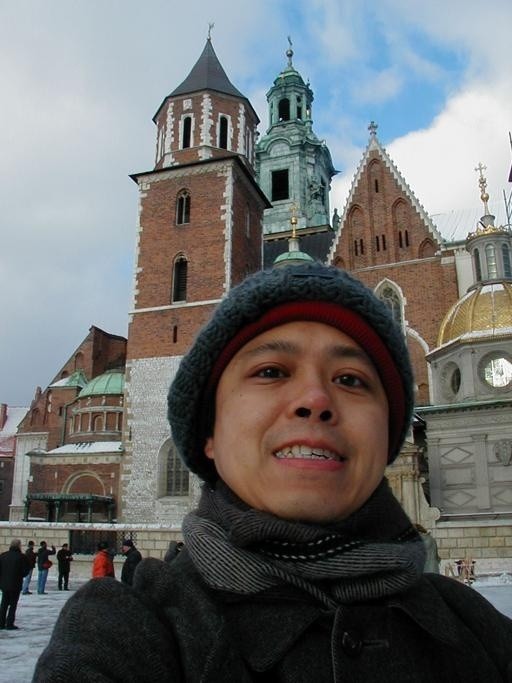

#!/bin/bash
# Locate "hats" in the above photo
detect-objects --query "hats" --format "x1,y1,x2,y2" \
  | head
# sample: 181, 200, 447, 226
198, 300, 406, 459
122, 540, 133, 547
166, 264, 414, 484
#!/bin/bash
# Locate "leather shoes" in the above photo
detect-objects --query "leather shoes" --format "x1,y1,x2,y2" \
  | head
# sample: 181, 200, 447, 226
0, 625, 18, 630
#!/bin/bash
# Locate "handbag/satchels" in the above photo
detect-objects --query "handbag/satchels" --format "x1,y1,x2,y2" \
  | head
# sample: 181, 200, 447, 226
42, 560, 52, 569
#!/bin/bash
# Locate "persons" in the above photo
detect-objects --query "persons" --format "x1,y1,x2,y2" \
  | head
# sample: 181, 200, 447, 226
92, 541, 116, 579
121, 539, 142, 586
57, 543, 73, 591
38, 541, 56, 594
0, 538, 29, 630
22, 541, 38, 595
31, 257, 511, 682
